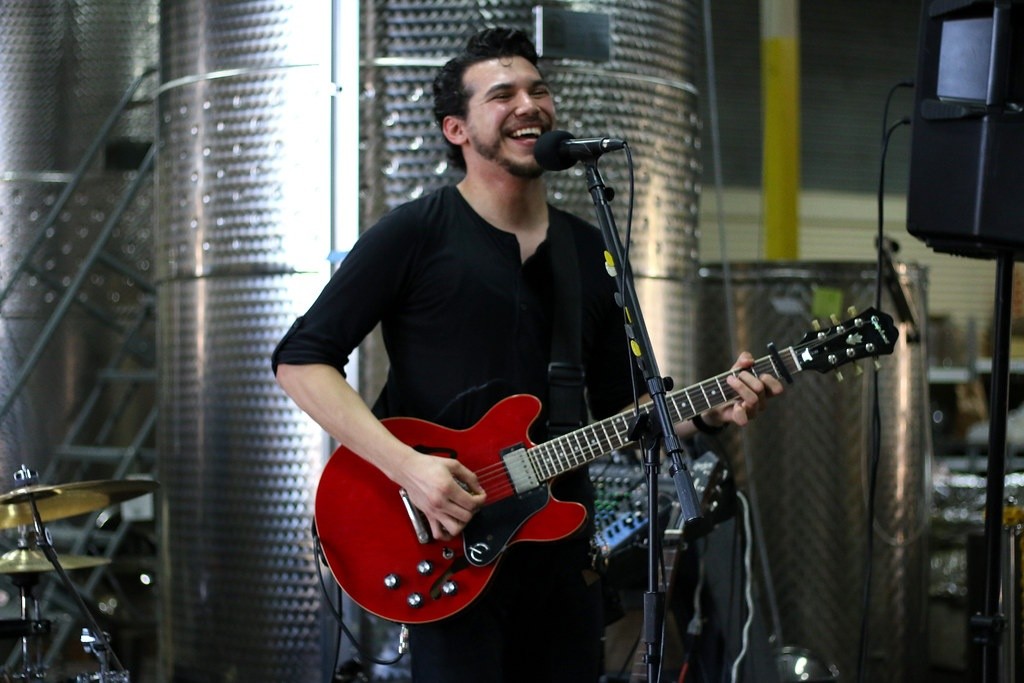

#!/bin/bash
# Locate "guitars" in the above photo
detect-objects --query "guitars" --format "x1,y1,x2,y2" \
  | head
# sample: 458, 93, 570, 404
305, 302, 901, 630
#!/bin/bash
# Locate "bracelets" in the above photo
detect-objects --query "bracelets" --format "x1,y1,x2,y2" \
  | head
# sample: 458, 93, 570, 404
693, 415, 731, 434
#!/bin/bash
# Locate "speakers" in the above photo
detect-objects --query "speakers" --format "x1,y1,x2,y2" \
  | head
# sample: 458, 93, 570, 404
906, 0, 1024, 262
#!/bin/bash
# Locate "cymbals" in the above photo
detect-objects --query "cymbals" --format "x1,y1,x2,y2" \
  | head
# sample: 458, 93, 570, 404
0, 539, 114, 577
0, 463, 162, 533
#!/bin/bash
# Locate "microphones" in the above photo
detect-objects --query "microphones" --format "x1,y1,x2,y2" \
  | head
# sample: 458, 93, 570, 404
532, 130, 627, 171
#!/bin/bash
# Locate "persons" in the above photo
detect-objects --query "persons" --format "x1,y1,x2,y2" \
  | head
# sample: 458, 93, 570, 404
269, 28, 784, 683
